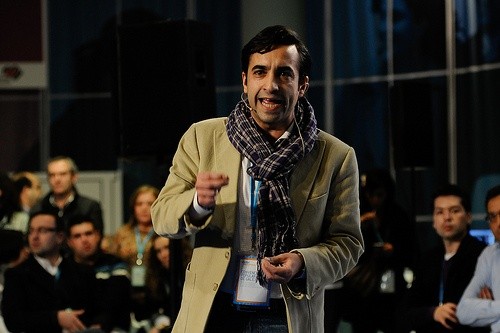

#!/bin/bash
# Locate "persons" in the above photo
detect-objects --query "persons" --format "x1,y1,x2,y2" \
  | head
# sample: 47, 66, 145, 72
148, 24, 365, 333
0, 158, 191, 333
324, 172, 500, 333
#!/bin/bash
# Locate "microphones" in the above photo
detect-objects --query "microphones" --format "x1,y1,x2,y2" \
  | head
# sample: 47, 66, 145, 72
240, 91, 253, 112
